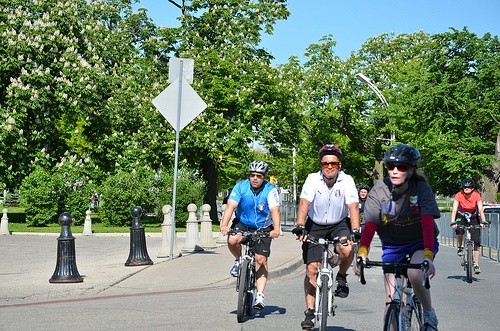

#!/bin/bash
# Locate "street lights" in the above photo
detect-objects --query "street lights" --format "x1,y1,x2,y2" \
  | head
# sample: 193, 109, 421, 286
278, 148, 298, 219
356, 73, 395, 146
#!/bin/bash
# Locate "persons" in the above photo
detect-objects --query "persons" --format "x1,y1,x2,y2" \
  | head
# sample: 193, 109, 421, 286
357, 184, 370, 215
220, 160, 281, 308
296, 144, 360, 327
353, 144, 441, 331
450, 178, 489, 272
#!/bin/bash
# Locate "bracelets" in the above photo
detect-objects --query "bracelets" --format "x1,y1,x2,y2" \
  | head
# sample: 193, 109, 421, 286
358, 247, 368, 256
424, 250, 433, 260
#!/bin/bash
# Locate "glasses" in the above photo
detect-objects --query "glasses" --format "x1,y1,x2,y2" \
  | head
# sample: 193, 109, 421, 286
250, 174, 264, 178
386, 161, 413, 172
321, 162, 339, 169
463, 186, 473, 189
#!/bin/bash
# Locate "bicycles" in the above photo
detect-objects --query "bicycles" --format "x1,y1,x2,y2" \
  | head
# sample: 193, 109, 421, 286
295, 232, 360, 331
450, 220, 492, 283
355, 257, 430, 331
223, 229, 274, 323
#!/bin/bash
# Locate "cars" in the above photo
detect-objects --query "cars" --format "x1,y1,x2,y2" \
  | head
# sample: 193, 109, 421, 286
482, 201, 500, 221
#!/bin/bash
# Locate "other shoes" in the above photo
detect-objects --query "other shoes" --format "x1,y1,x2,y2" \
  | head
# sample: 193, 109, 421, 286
336, 272, 349, 298
301, 308, 315, 327
474, 264, 481, 272
457, 245, 464, 255
423, 311, 438, 331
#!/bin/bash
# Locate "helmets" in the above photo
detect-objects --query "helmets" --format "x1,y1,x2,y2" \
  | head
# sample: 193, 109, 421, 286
319, 144, 342, 160
383, 144, 420, 161
247, 160, 269, 174
358, 185, 370, 192
462, 178, 474, 187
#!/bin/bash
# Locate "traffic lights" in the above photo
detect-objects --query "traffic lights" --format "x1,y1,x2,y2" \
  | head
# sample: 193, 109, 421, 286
272, 175, 276, 184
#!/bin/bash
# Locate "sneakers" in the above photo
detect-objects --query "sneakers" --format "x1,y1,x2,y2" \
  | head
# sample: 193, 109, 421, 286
253, 293, 265, 308
230, 261, 239, 274
